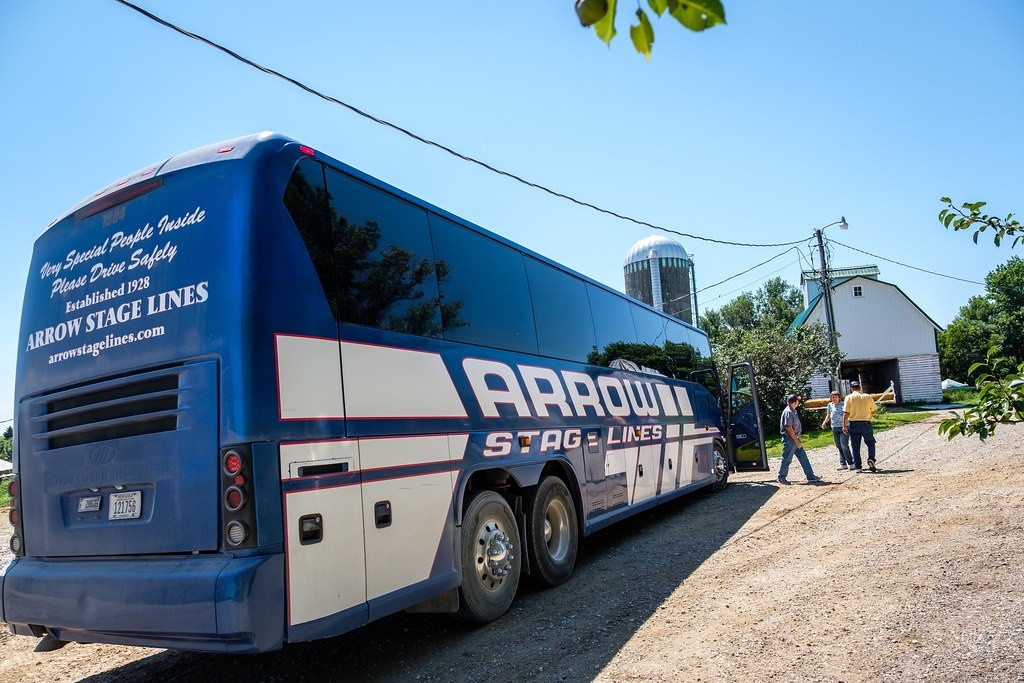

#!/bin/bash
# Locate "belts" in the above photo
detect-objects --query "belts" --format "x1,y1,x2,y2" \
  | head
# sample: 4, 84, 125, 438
850, 421, 870, 424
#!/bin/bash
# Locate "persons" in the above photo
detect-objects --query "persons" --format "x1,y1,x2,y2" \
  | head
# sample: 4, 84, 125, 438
776, 395, 823, 485
602, 410, 667, 449
821, 391, 855, 470
842, 381, 877, 473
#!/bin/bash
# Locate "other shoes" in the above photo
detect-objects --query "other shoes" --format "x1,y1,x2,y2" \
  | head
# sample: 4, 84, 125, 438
848, 464, 856, 470
806, 475, 823, 483
777, 474, 794, 485
867, 459, 877, 473
856, 469, 862, 473
836, 465, 848, 470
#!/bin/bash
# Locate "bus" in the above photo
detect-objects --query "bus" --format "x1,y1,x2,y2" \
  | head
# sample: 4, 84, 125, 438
0, 127, 770, 657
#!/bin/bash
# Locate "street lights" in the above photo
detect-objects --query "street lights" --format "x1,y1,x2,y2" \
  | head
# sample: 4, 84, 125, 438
821, 216, 849, 394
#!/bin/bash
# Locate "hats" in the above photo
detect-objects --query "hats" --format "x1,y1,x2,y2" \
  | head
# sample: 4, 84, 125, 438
850, 381, 860, 389
787, 394, 801, 404
